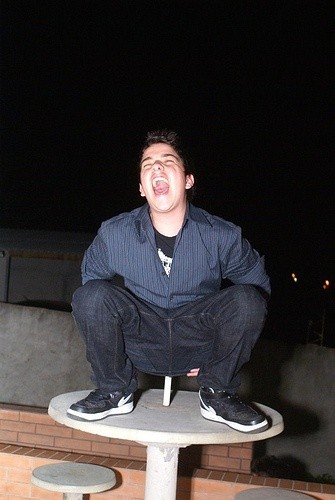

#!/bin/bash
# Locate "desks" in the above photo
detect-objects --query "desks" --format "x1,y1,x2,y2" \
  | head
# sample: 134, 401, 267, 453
47, 381, 285, 500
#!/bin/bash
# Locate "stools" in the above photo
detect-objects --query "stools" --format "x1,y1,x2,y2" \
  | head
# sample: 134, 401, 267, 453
233, 484, 319, 500
31, 460, 117, 500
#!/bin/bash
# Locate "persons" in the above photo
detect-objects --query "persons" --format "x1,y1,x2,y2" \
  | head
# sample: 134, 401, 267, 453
65, 130, 270, 434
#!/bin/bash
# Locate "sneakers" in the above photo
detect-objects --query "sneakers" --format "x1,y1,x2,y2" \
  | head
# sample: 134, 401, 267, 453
67, 389, 133, 422
199, 387, 270, 434
